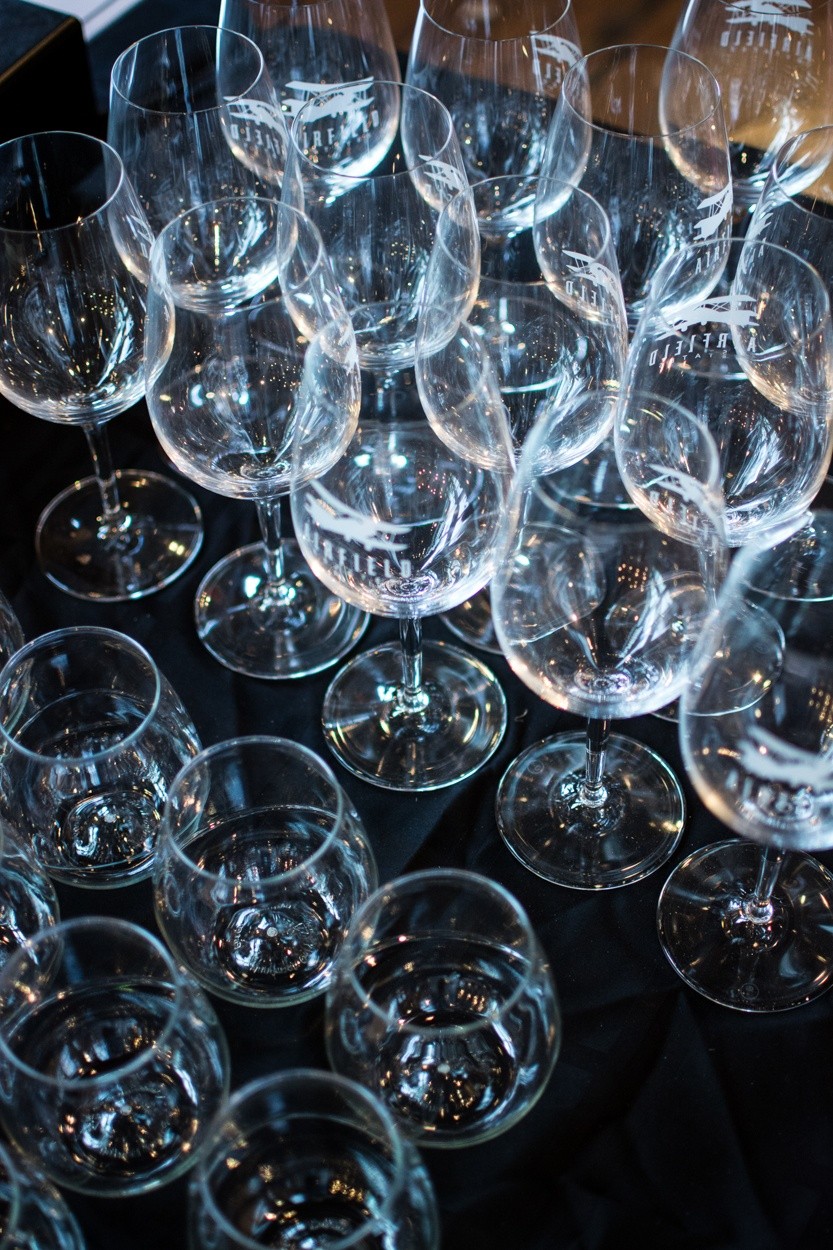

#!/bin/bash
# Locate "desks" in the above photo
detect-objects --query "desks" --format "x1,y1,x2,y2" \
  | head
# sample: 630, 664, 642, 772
1, 1, 833, 1250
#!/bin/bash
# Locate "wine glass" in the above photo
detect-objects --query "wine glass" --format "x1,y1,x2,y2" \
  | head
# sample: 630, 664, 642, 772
0, 0, 833, 793
652, 510, 833, 1012
497, 390, 730, 890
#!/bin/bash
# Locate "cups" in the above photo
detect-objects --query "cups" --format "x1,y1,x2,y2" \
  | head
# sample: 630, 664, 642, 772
1, 917, 230, 1196
0, 815, 59, 967
151, 736, 380, 1008
1, 627, 207, 889
0, 1132, 86, 1250
187, 1068, 439, 1250
326, 865, 563, 1148
